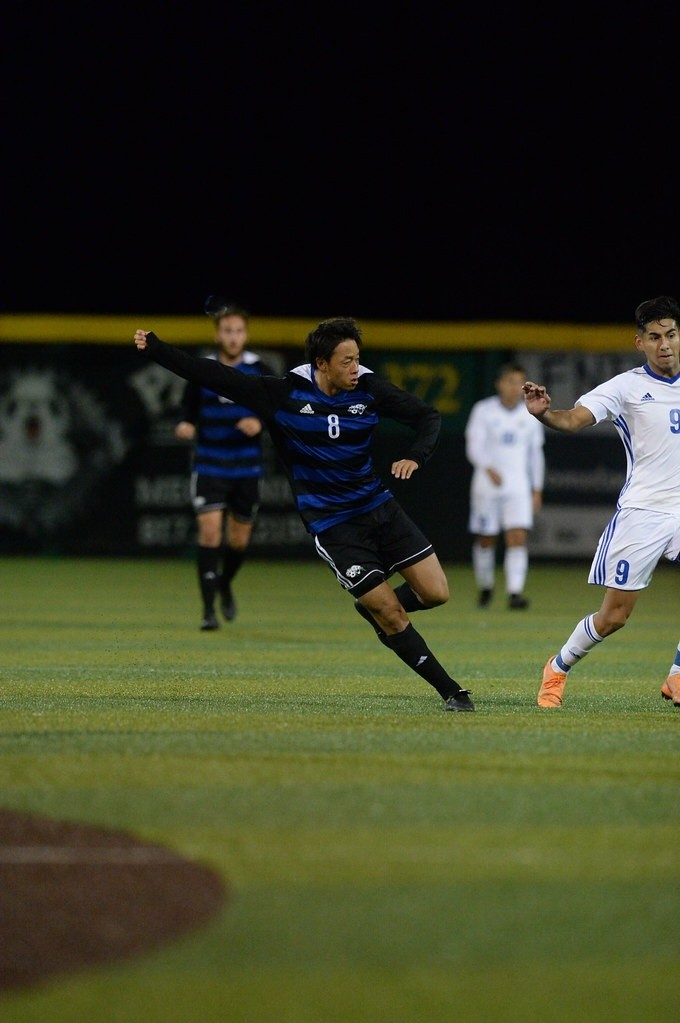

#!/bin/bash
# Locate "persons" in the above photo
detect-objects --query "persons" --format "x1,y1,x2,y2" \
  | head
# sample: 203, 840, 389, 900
131, 316, 478, 711
524, 295, 680, 708
466, 364, 548, 607
175, 305, 283, 633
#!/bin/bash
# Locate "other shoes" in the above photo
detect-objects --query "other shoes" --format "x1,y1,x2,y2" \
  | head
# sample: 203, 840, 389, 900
200, 610, 219, 630
220, 600, 236, 621
508, 593, 528, 607
478, 591, 490, 609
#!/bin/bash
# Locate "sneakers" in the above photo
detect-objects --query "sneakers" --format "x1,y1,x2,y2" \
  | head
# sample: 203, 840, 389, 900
537, 655, 567, 708
354, 599, 393, 649
446, 689, 475, 712
661, 673, 680, 708
